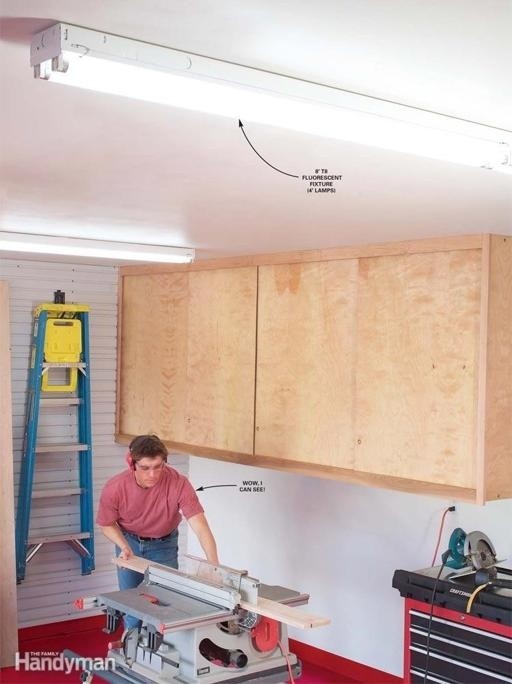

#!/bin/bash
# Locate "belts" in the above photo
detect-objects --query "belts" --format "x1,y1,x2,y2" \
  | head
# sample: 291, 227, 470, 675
137, 534, 171, 542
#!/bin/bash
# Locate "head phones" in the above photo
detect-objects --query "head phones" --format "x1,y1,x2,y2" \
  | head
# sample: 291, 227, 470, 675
125, 435, 168, 472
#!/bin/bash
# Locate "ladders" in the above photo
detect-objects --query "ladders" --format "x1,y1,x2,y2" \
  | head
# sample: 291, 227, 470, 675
16, 304, 95, 584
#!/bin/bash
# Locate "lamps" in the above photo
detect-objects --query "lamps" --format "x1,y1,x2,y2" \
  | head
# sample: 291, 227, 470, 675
30, 23, 512, 175
0, 230, 195, 265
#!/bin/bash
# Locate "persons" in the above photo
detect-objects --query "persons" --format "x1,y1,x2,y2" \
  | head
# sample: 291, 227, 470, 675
96, 434, 220, 634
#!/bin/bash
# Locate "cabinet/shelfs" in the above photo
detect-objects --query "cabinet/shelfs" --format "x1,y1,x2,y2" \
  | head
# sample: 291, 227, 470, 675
256, 232, 512, 506
115, 256, 255, 466
